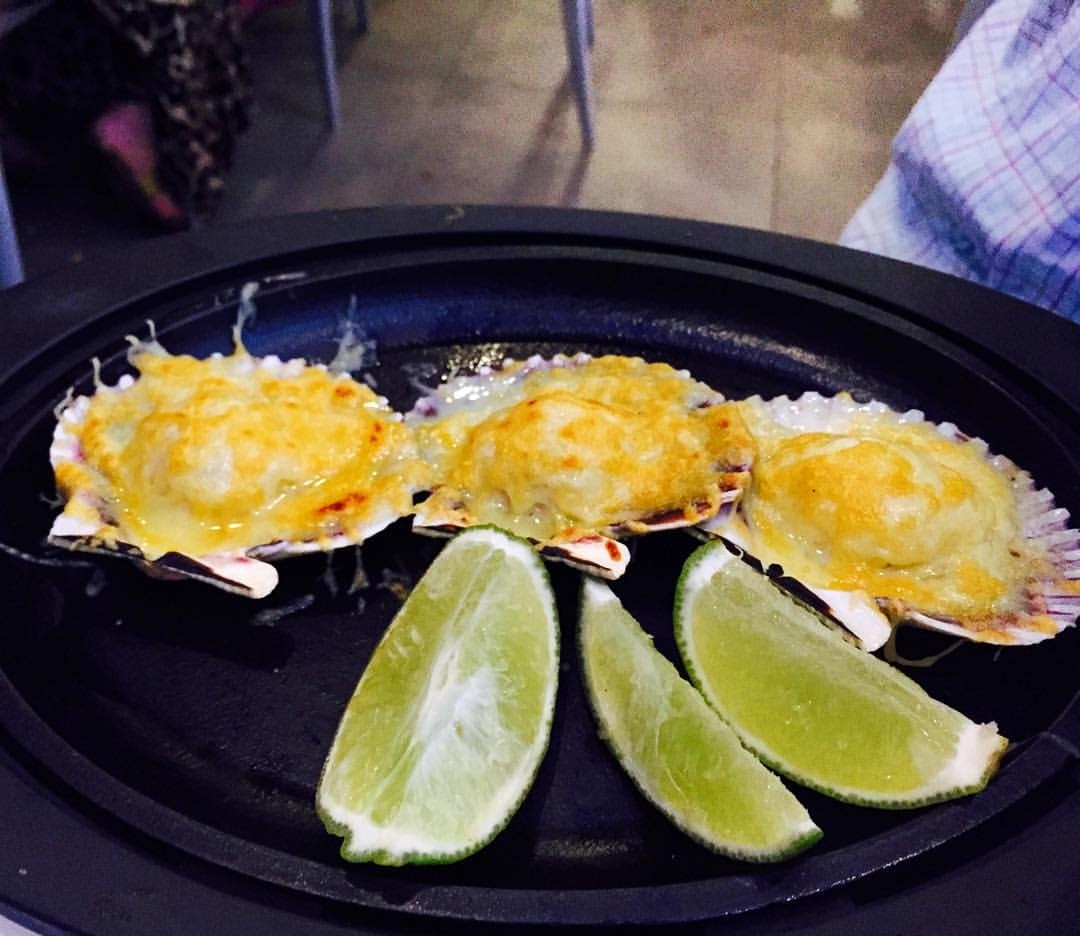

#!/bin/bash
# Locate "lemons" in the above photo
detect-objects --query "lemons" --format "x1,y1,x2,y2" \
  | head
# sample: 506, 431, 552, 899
581, 578, 824, 862
674, 538, 1009, 805
315, 525, 562, 865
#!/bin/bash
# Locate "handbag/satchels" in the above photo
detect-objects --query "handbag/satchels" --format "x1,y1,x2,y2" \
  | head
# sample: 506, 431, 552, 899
43, 0, 245, 225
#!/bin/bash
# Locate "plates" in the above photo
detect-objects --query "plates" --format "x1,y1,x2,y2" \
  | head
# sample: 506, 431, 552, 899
0, 238, 1080, 936
